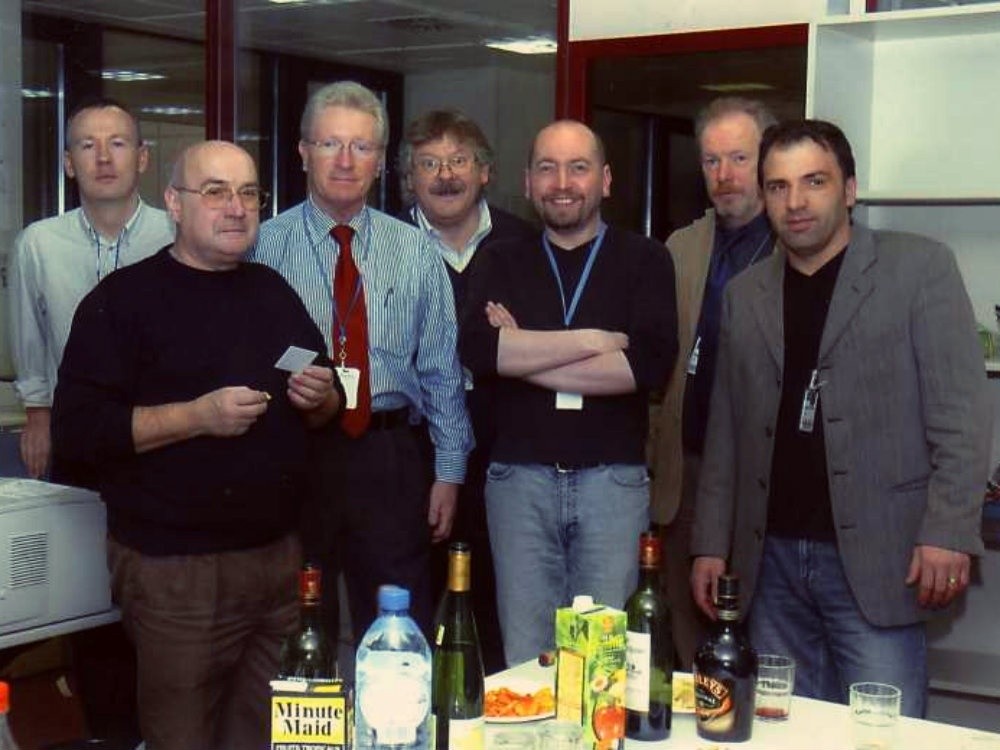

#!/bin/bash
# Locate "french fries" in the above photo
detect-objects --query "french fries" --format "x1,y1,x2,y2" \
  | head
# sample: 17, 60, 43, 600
485, 687, 555, 718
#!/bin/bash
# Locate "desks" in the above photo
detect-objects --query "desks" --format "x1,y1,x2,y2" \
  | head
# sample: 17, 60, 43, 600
480, 648, 1000, 750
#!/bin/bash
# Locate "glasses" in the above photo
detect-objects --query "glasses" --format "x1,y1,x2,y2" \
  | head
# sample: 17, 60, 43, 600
172, 183, 269, 212
422, 157, 466, 174
304, 137, 381, 160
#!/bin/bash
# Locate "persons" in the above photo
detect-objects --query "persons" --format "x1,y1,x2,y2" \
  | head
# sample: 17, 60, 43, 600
249, 81, 475, 680
53, 139, 347, 750
649, 96, 783, 672
7, 103, 178, 750
394, 109, 534, 677
688, 120, 990, 718
461, 117, 680, 666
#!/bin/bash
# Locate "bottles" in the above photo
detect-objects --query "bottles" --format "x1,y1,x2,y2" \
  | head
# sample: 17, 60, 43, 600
355, 586, 433, 750
281, 562, 338, 680
693, 576, 759, 744
432, 543, 485, 749
623, 530, 673, 742
1, 681, 20, 750
552, 595, 627, 750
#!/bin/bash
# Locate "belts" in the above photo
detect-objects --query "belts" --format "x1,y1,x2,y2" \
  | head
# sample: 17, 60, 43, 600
534, 462, 609, 473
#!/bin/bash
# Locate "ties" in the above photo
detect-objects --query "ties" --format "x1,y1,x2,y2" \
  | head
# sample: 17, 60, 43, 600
326, 229, 373, 440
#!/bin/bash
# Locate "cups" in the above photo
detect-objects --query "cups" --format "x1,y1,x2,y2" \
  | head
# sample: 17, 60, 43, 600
850, 681, 902, 749
753, 654, 797, 723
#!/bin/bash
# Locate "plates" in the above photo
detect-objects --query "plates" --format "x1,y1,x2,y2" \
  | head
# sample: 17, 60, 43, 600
484, 678, 555, 724
671, 671, 697, 715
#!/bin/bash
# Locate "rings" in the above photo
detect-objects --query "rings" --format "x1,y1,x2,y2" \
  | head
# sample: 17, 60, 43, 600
948, 576, 957, 584
